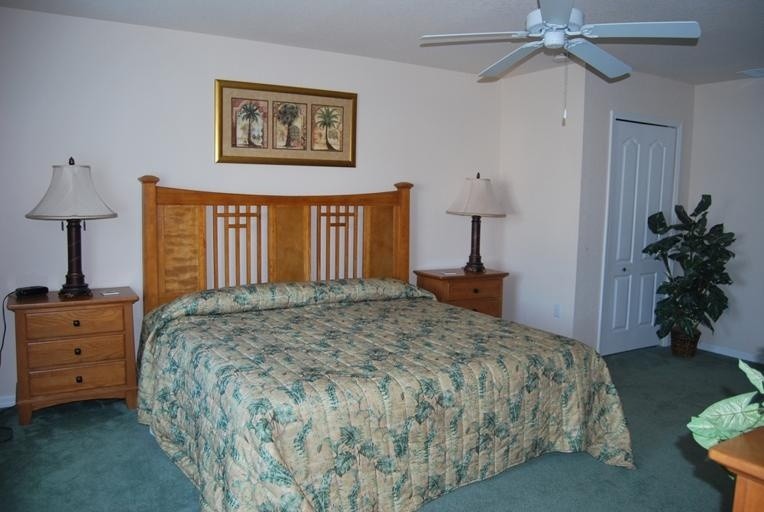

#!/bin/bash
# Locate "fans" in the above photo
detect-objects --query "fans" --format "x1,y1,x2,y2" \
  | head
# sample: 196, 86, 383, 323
419, 0, 700, 80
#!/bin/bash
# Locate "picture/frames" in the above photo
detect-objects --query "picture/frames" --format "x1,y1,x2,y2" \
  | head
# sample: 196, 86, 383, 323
214, 79, 358, 168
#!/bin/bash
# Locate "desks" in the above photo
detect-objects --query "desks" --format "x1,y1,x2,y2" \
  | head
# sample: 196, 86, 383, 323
708, 425, 764, 512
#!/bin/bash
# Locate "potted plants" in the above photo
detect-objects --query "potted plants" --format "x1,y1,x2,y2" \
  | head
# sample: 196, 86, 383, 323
642, 194, 737, 358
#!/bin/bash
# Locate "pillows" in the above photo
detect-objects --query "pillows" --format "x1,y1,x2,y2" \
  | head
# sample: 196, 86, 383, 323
185, 277, 420, 315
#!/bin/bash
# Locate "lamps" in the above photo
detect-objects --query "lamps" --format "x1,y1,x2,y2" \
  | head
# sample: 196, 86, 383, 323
544, 29, 564, 49
446, 174, 506, 273
25, 157, 118, 300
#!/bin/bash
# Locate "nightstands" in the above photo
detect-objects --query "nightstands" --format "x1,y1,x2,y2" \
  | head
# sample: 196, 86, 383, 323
414, 267, 508, 319
6, 286, 139, 425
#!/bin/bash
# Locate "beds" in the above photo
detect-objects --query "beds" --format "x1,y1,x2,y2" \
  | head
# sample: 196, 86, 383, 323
136, 173, 635, 512
